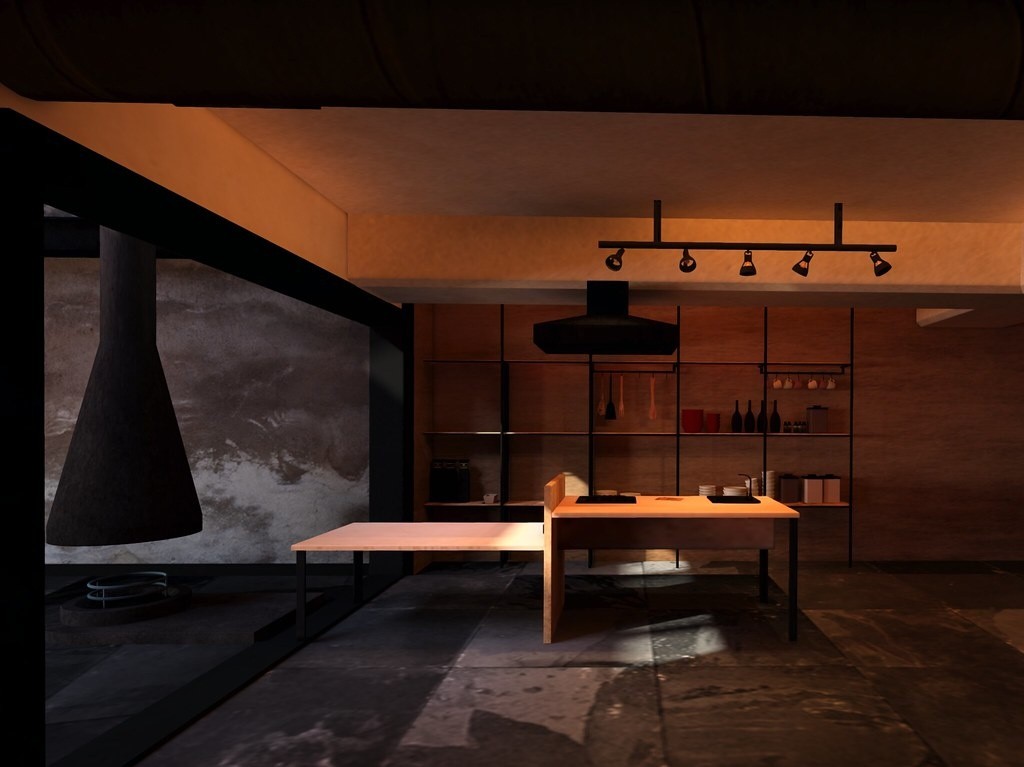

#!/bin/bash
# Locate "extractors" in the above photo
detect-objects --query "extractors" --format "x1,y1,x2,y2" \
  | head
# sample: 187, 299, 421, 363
533, 281, 678, 356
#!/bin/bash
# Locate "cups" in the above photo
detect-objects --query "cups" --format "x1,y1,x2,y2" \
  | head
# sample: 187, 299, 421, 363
827, 379, 836, 389
681, 409, 704, 433
819, 380, 826, 388
808, 379, 817, 389
705, 411, 720, 433
783, 378, 793, 389
794, 379, 802, 388
773, 379, 782, 388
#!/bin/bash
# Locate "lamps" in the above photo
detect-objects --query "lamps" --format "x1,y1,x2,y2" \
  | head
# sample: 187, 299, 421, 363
870, 251, 892, 277
740, 250, 756, 276
679, 248, 696, 273
792, 250, 814, 277
605, 249, 625, 272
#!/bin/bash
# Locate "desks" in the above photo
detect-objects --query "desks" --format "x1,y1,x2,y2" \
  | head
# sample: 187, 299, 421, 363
543, 474, 801, 644
290, 521, 543, 646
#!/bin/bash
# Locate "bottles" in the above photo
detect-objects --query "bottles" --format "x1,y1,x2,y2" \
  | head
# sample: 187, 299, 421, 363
456, 458, 470, 502
757, 400, 768, 432
745, 400, 755, 433
430, 458, 445, 494
770, 400, 780, 432
732, 400, 742, 432
783, 421, 807, 433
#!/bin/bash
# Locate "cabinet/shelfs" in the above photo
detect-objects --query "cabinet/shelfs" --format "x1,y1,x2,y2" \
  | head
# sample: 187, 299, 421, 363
423, 306, 855, 568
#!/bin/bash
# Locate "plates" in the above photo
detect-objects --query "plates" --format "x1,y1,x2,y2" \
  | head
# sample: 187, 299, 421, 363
698, 485, 716, 496
723, 486, 746, 496
745, 478, 759, 496
762, 471, 774, 498
